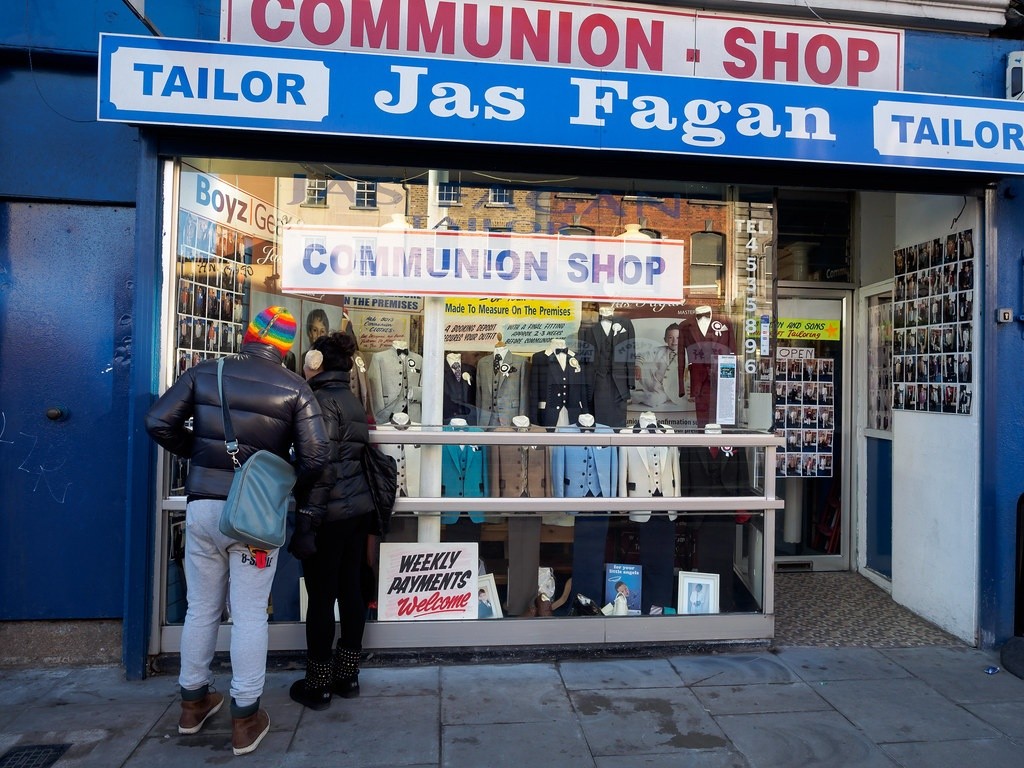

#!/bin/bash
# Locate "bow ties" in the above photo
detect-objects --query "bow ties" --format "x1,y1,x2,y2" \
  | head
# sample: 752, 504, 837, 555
599, 314, 613, 323
397, 349, 408, 356
556, 347, 568, 354
696, 312, 710, 320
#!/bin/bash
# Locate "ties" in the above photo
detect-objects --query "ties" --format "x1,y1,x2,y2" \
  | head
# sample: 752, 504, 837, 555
697, 592, 698, 607
664, 607, 675, 614
709, 447, 718, 459
487, 600, 491, 606
493, 354, 502, 376
451, 362, 461, 383
649, 605, 662, 614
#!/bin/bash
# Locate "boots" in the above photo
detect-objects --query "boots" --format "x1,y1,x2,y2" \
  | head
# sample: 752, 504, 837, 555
230, 697, 270, 756
335, 638, 362, 699
178, 678, 224, 736
290, 655, 335, 711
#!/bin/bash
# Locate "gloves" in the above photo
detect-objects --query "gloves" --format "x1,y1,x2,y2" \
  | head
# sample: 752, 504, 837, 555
287, 504, 328, 560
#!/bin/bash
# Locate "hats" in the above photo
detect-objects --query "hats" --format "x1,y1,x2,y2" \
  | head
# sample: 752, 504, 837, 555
242, 306, 297, 360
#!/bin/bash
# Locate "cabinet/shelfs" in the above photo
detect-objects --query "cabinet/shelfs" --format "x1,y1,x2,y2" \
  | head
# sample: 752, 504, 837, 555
441, 523, 575, 611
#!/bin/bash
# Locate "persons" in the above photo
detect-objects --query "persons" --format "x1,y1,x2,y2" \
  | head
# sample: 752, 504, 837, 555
350, 307, 754, 619
760, 357, 834, 477
615, 581, 640, 610
690, 583, 705, 613
678, 305, 737, 434
147, 306, 329, 754
627, 323, 696, 406
305, 310, 329, 343
892, 232, 972, 413
288, 332, 397, 707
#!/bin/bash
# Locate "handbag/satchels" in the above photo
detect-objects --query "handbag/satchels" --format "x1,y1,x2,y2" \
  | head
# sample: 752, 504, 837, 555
217, 357, 297, 550
361, 442, 397, 541
613, 592, 628, 615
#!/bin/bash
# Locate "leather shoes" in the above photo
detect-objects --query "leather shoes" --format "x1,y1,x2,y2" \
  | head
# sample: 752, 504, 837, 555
535, 593, 552, 616
521, 596, 538, 617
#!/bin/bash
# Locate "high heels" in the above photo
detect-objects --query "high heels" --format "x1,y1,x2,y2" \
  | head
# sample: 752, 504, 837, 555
572, 592, 599, 616
551, 576, 575, 616
